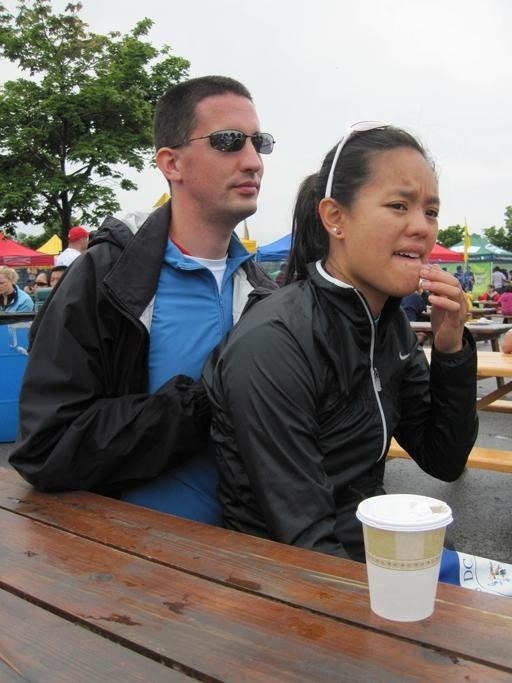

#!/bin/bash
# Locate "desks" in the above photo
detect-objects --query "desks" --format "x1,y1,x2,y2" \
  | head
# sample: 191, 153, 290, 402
468, 307, 496, 315
409, 320, 511, 390
474, 300, 499, 307
1, 461, 512, 681
421, 347, 511, 413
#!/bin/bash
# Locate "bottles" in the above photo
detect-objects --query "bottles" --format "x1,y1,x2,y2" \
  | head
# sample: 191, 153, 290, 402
356, 491, 454, 622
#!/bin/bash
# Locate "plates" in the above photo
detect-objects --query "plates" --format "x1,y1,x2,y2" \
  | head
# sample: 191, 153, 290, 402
467, 320, 492, 324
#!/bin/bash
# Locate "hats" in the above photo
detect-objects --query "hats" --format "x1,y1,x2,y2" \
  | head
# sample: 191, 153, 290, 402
66, 225, 91, 242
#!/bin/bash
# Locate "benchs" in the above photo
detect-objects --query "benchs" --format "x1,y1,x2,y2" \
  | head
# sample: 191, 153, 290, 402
384, 437, 511, 474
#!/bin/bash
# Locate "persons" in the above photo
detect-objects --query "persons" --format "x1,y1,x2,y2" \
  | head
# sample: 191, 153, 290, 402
276, 263, 287, 286
0, 226, 94, 314
208, 124, 476, 565
403, 264, 512, 324
7, 75, 277, 528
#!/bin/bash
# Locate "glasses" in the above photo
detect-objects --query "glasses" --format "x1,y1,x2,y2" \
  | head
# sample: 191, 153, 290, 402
34, 280, 50, 287
323, 118, 437, 202
161, 127, 278, 155
